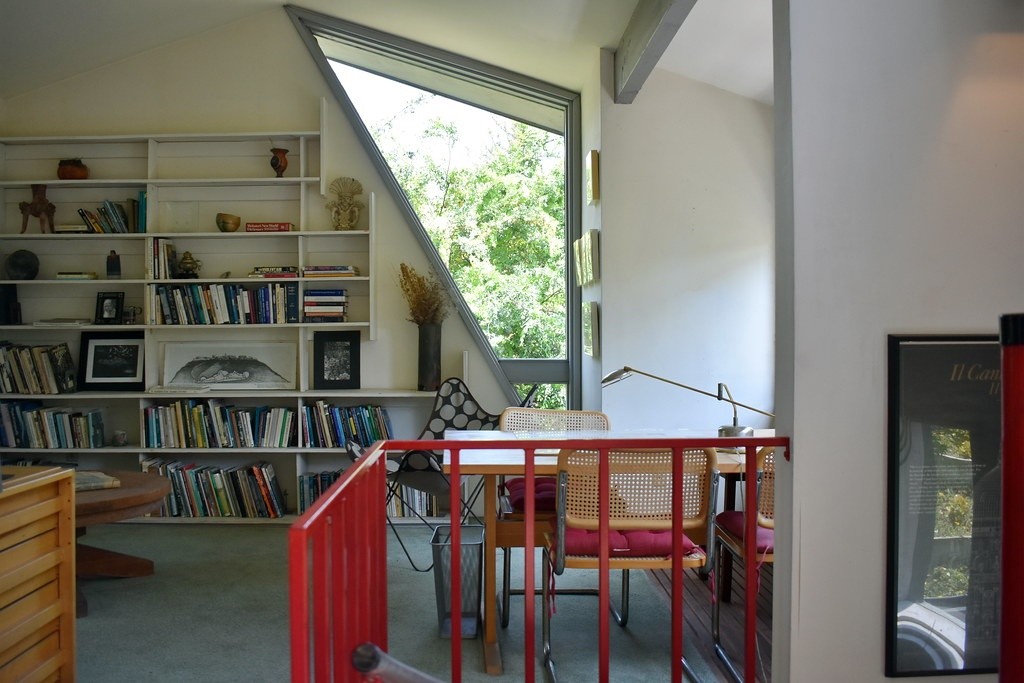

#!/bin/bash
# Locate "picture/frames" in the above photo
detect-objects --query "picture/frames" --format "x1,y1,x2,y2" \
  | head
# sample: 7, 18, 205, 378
76, 331, 146, 391
164, 343, 297, 390
95, 291, 125, 325
313, 330, 361, 392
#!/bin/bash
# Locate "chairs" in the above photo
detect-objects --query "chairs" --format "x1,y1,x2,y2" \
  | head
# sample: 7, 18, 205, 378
496, 407, 776, 683
345, 378, 539, 571
542, 446, 776, 683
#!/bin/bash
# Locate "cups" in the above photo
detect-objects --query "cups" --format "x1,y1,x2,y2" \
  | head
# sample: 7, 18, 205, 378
122, 306, 136, 325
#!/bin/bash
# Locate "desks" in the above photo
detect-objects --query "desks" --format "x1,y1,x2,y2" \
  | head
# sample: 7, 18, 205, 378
443, 430, 780, 677
73, 470, 170, 578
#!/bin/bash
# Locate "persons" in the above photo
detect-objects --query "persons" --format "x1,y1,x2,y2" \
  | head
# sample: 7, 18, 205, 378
105, 299, 115, 318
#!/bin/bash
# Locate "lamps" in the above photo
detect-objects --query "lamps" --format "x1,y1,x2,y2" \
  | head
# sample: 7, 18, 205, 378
600, 366, 774, 419
716, 382, 754, 438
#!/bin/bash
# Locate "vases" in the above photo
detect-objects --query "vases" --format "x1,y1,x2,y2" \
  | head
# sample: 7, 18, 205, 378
270, 147, 289, 179
418, 323, 442, 392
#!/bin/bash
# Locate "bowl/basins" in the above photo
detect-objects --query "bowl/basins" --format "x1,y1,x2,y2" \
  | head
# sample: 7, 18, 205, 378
57, 164, 88, 179
216, 212, 241, 231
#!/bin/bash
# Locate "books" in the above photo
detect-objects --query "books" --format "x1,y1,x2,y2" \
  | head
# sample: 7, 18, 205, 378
248, 266, 299, 279
302, 400, 395, 449
302, 289, 349, 323
54, 190, 147, 234
33, 318, 91, 326
147, 237, 179, 280
14, 459, 40, 467
142, 457, 287, 518
0, 404, 104, 449
301, 266, 361, 277
147, 283, 299, 325
55, 271, 100, 279
144, 399, 295, 448
299, 469, 441, 518
246, 222, 296, 233
0, 340, 78, 395
75, 471, 122, 493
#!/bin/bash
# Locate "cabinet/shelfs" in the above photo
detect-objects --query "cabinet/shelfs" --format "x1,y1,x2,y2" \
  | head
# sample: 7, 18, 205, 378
0, 464, 78, 683
0, 98, 471, 524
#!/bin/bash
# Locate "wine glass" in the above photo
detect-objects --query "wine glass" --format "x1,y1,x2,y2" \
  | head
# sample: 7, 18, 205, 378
270, 147, 289, 177
